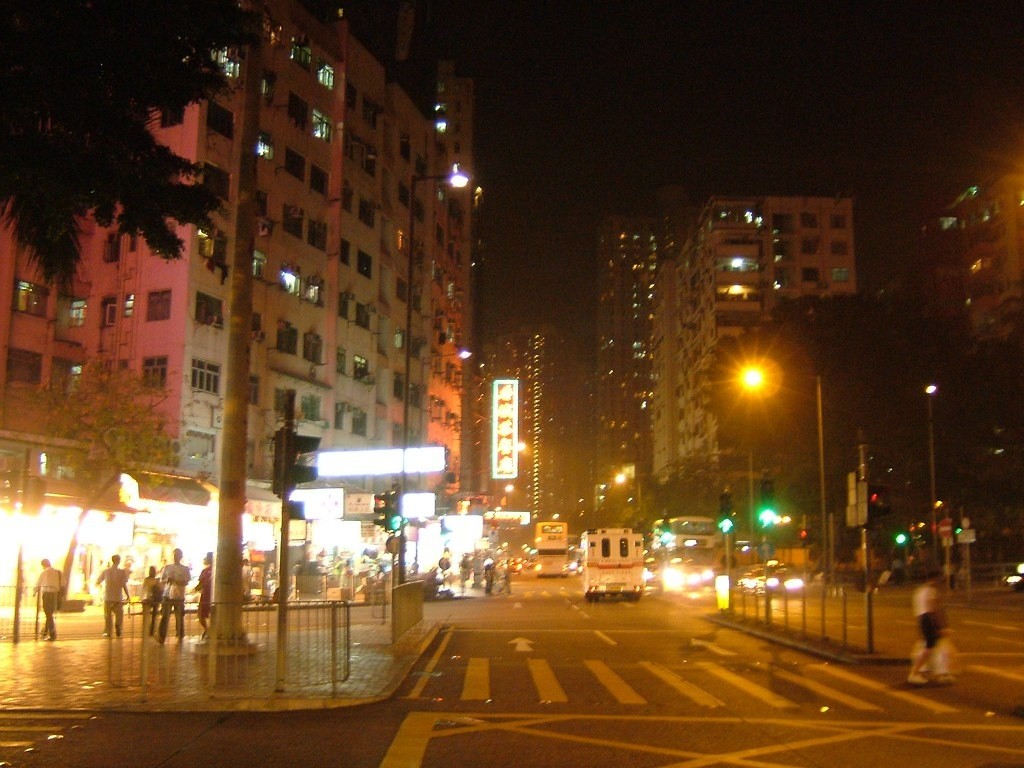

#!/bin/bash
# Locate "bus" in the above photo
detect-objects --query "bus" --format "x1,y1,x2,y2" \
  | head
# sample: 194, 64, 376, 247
534, 522, 569, 579
651, 516, 716, 567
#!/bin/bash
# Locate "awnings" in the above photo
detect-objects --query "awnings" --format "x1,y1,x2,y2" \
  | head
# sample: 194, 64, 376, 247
20, 490, 140, 514
206, 480, 282, 523
128, 471, 211, 507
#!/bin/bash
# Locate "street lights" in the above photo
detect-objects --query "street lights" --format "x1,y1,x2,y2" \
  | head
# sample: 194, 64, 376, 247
924, 376, 939, 580
733, 335, 830, 591
395, 163, 470, 581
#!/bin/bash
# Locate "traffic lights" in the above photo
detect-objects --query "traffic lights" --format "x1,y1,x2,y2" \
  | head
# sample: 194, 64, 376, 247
761, 481, 776, 526
719, 493, 733, 532
894, 531, 908, 547
869, 481, 879, 508
952, 508, 965, 535
289, 433, 321, 487
799, 528, 808, 539
389, 492, 402, 531
373, 493, 390, 532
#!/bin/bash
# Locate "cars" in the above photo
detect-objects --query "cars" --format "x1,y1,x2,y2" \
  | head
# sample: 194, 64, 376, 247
733, 562, 805, 600
499, 546, 584, 575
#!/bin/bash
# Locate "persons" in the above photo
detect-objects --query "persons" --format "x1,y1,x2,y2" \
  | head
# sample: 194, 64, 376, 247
194, 552, 213, 639
123, 563, 131, 581
411, 556, 419, 573
439, 547, 454, 588
908, 570, 956, 685
140, 565, 161, 637
484, 555, 495, 595
459, 553, 482, 595
497, 559, 512, 595
154, 549, 191, 645
944, 561, 956, 589
242, 559, 250, 595
33, 558, 67, 641
892, 557, 904, 585
340, 558, 352, 602
96, 554, 129, 636
265, 562, 276, 593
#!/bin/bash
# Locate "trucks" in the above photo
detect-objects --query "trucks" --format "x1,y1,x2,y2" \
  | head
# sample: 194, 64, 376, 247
579, 527, 646, 601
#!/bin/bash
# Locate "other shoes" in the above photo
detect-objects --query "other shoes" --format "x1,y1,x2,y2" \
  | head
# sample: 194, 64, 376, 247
907, 674, 928, 684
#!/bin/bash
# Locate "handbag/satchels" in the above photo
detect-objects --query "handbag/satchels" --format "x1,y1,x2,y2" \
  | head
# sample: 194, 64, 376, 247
932, 638, 964, 676
909, 640, 926, 662
147, 578, 163, 601
